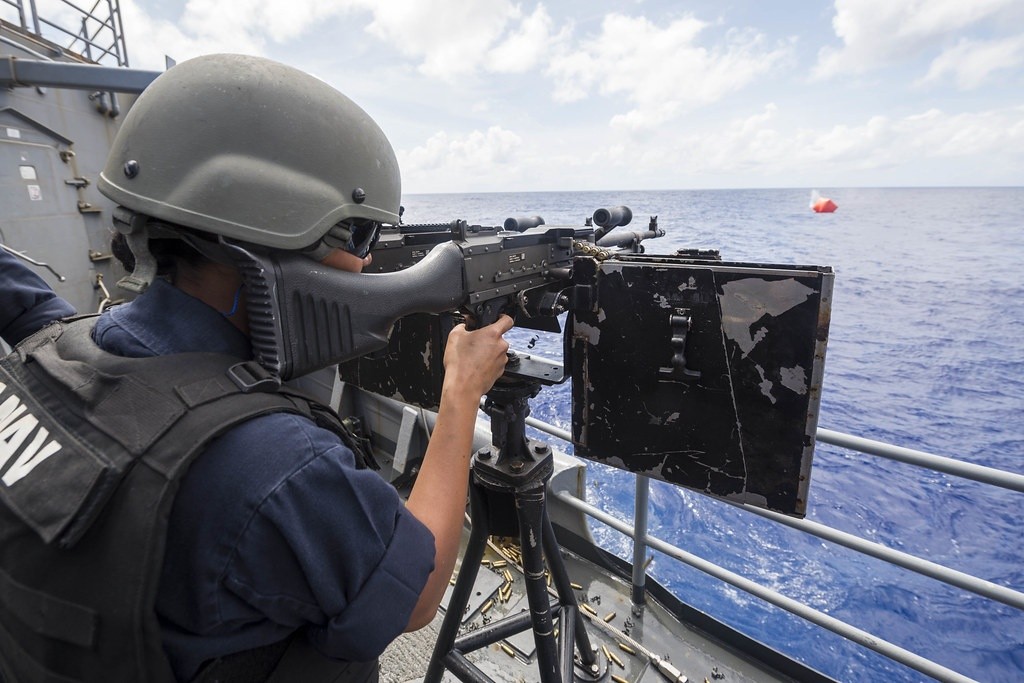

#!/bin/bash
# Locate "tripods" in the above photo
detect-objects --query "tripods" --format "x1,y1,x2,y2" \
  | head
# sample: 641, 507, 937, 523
424, 344, 595, 683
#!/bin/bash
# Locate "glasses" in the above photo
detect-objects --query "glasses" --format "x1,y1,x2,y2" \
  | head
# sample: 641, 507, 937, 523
333, 219, 382, 259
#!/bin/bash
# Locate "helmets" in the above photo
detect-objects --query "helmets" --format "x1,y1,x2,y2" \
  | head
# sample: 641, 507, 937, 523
98, 52, 402, 252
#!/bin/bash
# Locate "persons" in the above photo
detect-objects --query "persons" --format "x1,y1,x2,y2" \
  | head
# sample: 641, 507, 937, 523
0, 56, 514, 683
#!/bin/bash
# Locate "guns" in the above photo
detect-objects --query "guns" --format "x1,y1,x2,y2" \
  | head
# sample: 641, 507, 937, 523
239, 205, 666, 409
